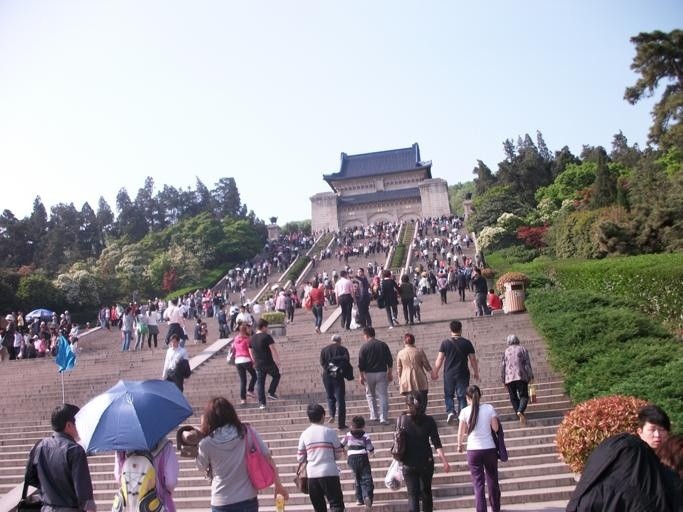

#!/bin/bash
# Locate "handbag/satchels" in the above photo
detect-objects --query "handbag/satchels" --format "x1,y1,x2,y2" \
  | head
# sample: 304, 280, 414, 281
18, 487, 42, 511
391, 427, 418, 465
529, 387, 536, 403
305, 296, 312, 310
138, 323, 149, 334
294, 461, 309, 494
226, 344, 236, 364
355, 311, 362, 324
377, 294, 386, 308
244, 441, 275, 490
328, 366, 344, 378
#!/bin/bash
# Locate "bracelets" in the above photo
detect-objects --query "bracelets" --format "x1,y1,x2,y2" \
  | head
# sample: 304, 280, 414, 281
458, 444, 463, 448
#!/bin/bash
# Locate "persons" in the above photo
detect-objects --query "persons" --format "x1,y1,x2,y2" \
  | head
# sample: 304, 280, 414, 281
653, 434, 682, 512
565, 433, 683, 512
297, 404, 346, 512
501, 335, 533, 426
196, 395, 289, 512
430, 321, 480, 423
222, 215, 503, 334
395, 391, 450, 512
231, 319, 282, 409
0, 309, 79, 372
98, 287, 229, 351
320, 335, 350, 429
162, 334, 187, 396
634, 406, 669, 453
457, 384, 500, 512
26, 404, 95, 512
396, 333, 433, 396
339, 415, 375, 512
357, 326, 393, 425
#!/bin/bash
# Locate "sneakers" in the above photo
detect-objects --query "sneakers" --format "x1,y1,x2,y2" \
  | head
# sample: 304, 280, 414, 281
393, 317, 399, 324
447, 413, 455, 424
315, 326, 321, 334
259, 404, 265, 410
241, 399, 246, 404
247, 391, 256, 398
267, 393, 278, 400
381, 419, 389, 425
364, 496, 372, 511
341, 319, 344, 329
328, 417, 335, 424
517, 411, 525, 421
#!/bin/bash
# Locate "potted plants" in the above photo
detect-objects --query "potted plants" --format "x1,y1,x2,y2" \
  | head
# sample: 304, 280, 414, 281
497, 272, 529, 314
482, 269, 496, 289
261, 312, 286, 337
554, 395, 650, 482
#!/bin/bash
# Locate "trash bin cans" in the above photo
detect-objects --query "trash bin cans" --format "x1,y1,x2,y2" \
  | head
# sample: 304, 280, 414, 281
503, 281, 526, 314
